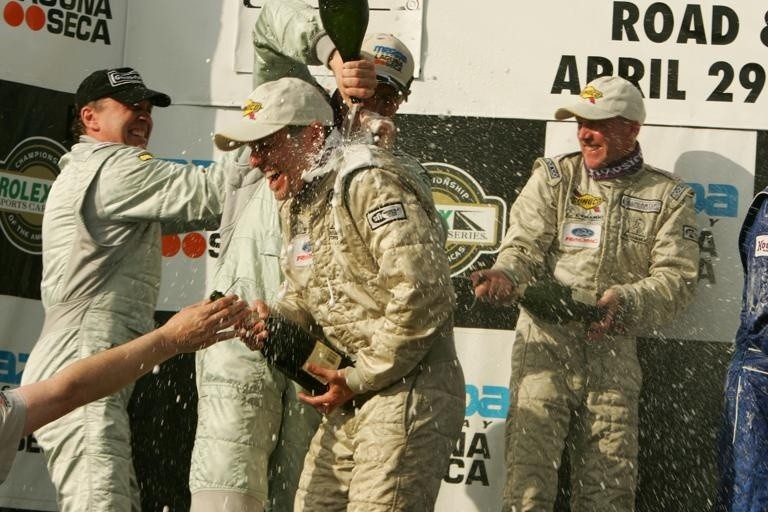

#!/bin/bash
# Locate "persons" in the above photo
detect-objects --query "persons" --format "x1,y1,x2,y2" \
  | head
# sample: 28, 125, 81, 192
469, 73, 701, 511
716, 184, 768, 511
210, 76, 468, 512
1, 293, 253, 485
19, 67, 225, 512
184, 1, 434, 512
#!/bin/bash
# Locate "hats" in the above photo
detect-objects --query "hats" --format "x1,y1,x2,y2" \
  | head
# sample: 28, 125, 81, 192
215, 77, 335, 150
555, 75, 646, 126
360, 33, 413, 94
75, 67, 171, 112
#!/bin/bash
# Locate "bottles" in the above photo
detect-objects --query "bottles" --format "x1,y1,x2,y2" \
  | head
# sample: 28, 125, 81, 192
477, 277, 604, 329
209, 290, 354, 402
318, 0, 369, 104
